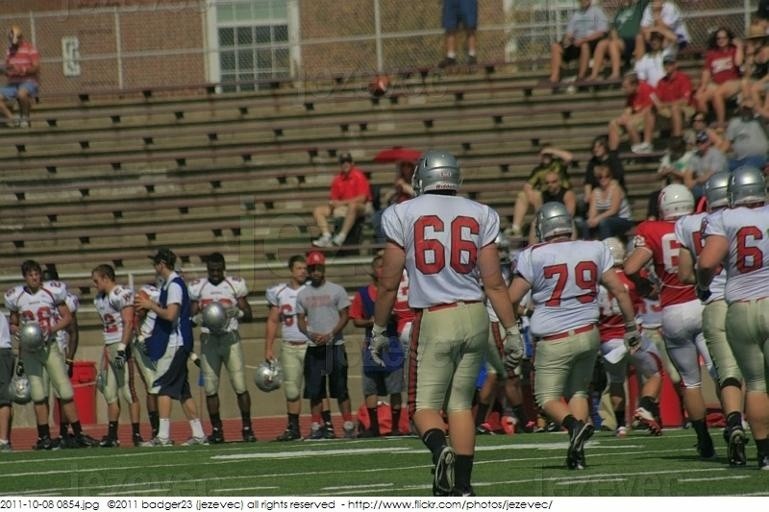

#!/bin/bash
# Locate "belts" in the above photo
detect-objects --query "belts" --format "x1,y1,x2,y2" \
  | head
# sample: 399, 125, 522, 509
290, 341, 306, 346
544, 324, 593, 340
428, 300, 482, 312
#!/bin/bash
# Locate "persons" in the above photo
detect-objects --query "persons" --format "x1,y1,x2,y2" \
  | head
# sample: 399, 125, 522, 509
537, 0, 769, 154
0, 24, 43, 129
1, 139, 769, 496
438, 0, 480, 66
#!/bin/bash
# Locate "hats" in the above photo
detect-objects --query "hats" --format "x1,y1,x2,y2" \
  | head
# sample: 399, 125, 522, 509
664, 54, 675, 63
340, 150, 352, 162
147, 246, 177, 266
305, 251, 328, 267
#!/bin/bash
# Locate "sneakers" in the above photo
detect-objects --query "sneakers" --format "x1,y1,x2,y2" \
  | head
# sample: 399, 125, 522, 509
635, 407, 662, 435
724, 425, 747, 466
631, 142, 654, 154
313, 234, 346, 246
697, 440, 718, 460
475, 411, 560, 435
565, 420, 594, 470
276, 427, 406, 441
33, 427, 257, 451
432, 441, 456, 495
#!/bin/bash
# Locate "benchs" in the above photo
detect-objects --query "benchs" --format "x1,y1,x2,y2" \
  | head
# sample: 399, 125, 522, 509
0, 42, 769, 349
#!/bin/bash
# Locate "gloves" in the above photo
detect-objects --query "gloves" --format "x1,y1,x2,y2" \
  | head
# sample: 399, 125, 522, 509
636, 276, 654, 295
503, 324, 523, 370
114, 341, 127, 370
368, 323, 391, 367
624, 323, 642, 355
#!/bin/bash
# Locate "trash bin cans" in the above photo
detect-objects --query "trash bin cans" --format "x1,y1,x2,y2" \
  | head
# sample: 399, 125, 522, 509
53, 362, 97, 427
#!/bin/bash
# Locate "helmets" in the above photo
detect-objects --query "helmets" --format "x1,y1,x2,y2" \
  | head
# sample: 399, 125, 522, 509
411, 150, 463, 193
707, 171, 731, 210
9, 375, 32, 404
18, 322, 45, 351
203, 302, 227, 331
254, 358, 282, 390
731, 165, 766, 207
658, 183, 694, 220
536, 202, 573, 239
